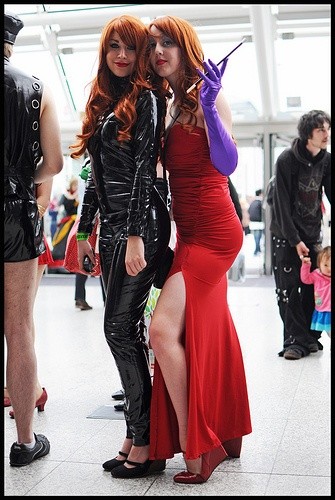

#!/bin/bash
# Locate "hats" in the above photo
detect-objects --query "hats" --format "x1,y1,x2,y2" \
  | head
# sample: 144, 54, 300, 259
4, 13, 24, 45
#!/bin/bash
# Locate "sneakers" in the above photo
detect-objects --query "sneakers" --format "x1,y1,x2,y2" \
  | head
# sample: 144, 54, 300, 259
10, 432, 50, 467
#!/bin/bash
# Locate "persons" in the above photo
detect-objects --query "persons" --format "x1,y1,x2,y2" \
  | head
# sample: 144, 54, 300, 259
4, 12, 51, 468
53, 177, 79, 259
63, 156, 107, 310
4, 174, 53, 416
270, 110, 332, 359
67, 14, 171, 478
247, 189, 265, 256
148, 16, 252, 484
300, 246, 331, 340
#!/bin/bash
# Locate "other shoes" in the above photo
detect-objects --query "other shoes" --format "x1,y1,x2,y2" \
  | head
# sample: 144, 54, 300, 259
280, 343, 318, 359
112, 389, 125, 410
75, 300, 92, 310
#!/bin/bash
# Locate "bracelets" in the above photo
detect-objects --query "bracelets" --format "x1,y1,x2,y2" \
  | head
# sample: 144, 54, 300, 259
76, 233, 90, 241
37, 203, 46, 216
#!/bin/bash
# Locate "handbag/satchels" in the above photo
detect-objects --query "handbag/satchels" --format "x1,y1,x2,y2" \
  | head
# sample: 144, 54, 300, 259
245, 226, 251, 235
229, 178, 242, 222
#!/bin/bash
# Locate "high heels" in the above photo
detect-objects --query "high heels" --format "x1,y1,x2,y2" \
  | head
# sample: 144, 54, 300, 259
174, 436, 242, 484
4, 387, 48, 418
103, 451, 166, 477
